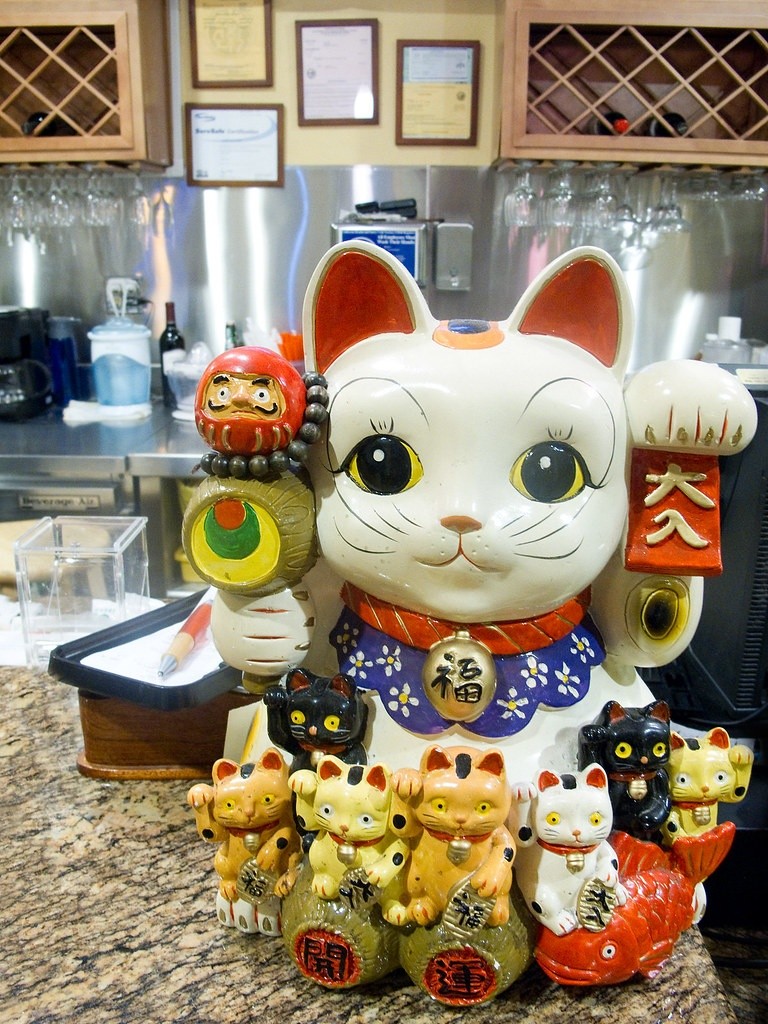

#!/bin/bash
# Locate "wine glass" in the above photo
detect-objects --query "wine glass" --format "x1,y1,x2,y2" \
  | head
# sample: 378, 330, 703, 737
0, 163, 151, 228
503, 159, 768, 231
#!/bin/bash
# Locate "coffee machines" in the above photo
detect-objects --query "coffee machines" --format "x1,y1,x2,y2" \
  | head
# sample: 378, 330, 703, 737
0, 306, 53, 420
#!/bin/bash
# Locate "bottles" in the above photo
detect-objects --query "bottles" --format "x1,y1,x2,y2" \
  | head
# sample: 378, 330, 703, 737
21, 111, 79, 136
159, 302, 185, 408
224, 323, 237, 351
642, 112, 688, 138
588, 112, 629, 136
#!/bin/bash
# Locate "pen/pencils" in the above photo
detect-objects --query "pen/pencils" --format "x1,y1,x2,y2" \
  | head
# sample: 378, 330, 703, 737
155, 599, 213, 677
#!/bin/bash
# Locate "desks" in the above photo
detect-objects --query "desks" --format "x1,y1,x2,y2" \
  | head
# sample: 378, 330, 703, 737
0, 406, 213, 604
